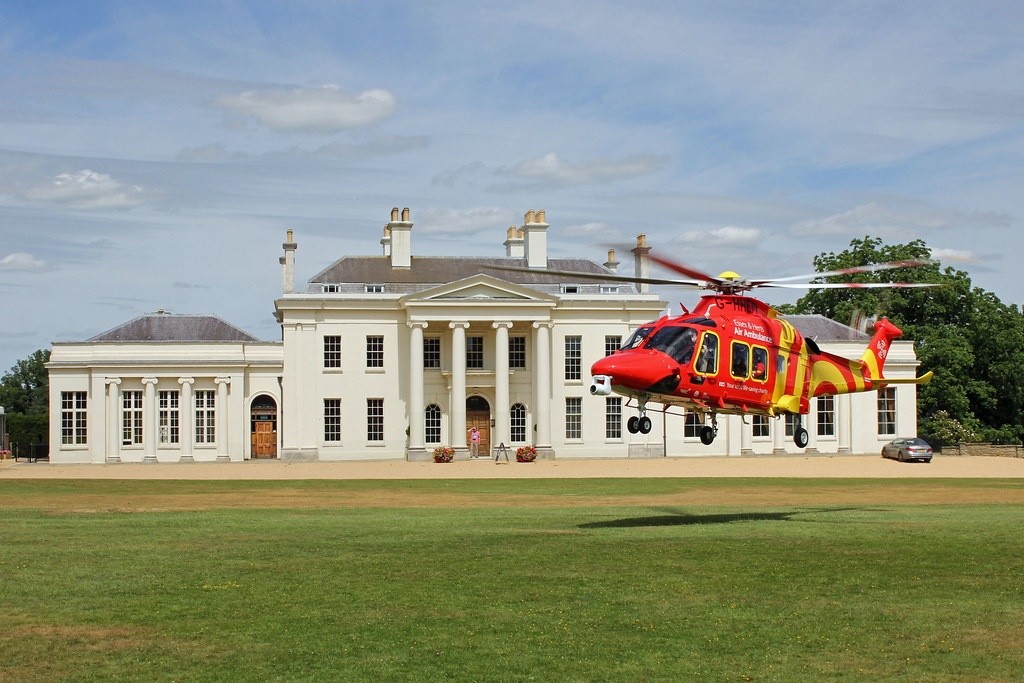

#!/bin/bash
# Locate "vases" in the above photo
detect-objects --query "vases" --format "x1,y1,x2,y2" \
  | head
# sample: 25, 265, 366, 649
435, 456, 451, 463
518, 456, 532, 462
0, 453, 10, 459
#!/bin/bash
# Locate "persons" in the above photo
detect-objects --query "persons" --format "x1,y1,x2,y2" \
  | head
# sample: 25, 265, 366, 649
675, 329, 710, 372
468, 427, 480, 458
752, 351, 765, 380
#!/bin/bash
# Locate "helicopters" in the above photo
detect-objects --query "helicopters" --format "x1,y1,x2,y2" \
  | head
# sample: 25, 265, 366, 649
476, 241, 952, 448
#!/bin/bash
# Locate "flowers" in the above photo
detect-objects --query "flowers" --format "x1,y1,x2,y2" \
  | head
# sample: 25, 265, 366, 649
515, 446, 538, 461
432, 446, 455, 461
0, 449, 11, 455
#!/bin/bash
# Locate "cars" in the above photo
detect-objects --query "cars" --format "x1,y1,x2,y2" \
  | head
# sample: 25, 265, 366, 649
881, 438, 933, 463
